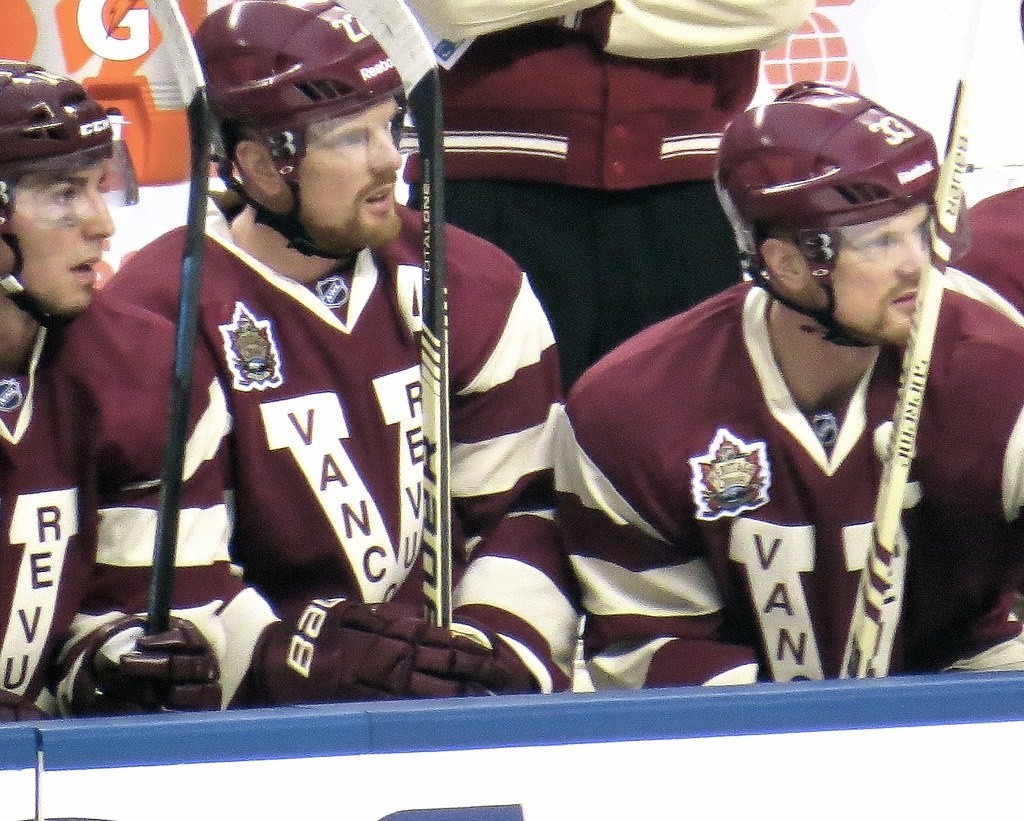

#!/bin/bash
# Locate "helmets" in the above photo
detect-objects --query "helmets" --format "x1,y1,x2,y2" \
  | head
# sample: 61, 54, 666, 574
0, 58, 112, 178
191, 0, 408, 184
715, 80, 941, 284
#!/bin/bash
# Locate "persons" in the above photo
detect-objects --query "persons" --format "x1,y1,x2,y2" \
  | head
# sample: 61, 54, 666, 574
56, 0, 583, 718
403, 0, 813, 404
0, 66, 225, 723
548, 82, 1024, 688
948, 183, 1024, 315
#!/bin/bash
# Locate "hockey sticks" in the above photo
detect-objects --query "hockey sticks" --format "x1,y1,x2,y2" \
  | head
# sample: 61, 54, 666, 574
141, 2, 214, 636
343, 0, 451, 627
837, 76, 974, 682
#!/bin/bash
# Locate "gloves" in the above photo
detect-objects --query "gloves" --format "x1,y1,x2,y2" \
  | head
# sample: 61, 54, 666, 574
250, 596, 494, 706
58, 612, 222, 718
